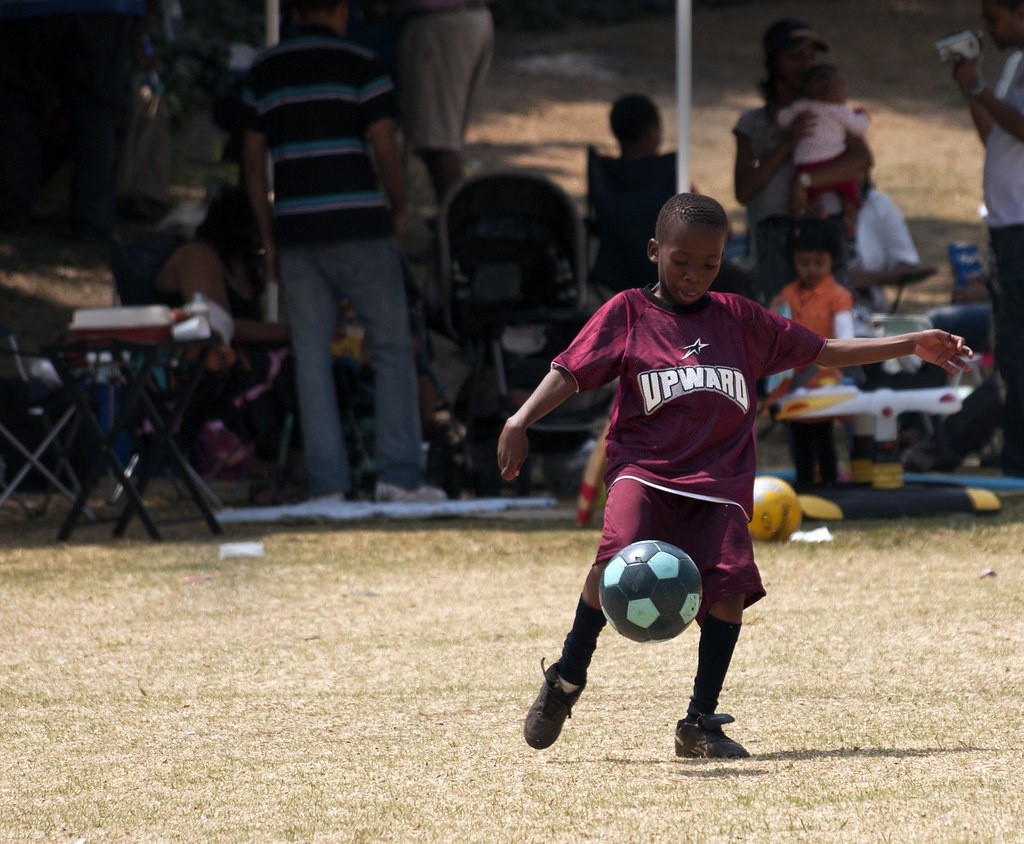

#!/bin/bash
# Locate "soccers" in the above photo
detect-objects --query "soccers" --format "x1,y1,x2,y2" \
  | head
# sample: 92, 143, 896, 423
597, 540, 705, 646
747, 473, 801, 543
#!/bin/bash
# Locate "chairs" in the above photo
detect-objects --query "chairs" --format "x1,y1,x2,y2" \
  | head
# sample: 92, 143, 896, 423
0, 320, 101, 523
584, 145, 678, 305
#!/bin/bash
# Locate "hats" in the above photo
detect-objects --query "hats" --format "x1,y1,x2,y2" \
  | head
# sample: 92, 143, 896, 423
765, 17, 832, 54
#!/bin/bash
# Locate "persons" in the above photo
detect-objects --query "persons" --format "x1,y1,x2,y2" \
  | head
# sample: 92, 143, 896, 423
496, 192, 973, 759
114, 0, 1024, 561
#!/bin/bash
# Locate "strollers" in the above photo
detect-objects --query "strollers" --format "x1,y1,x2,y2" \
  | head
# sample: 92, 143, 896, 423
423, 164, 618, 497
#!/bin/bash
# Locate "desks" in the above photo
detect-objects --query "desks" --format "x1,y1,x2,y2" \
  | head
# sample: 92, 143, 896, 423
44, 313, 295, 543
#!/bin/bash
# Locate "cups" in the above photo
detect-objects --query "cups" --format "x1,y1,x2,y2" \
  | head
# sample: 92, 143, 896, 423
934, 30, 984, 61
171, 316, 210, 340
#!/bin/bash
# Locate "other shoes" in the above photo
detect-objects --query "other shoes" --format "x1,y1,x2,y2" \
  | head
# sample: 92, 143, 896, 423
312, 493, 344, 501
375, 482, 448, 502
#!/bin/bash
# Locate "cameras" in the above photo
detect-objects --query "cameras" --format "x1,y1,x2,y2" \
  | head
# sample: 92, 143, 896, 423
936, 31, 982, 62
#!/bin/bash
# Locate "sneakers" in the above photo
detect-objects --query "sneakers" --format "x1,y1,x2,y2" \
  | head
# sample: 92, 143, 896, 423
675, 713, 750, 759
524, 658, 587, 749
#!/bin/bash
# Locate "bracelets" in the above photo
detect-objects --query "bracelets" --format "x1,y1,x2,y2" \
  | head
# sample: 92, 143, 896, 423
259, 247, 276, 253
960, 77, 985, 95
799, 170, 813, 188
